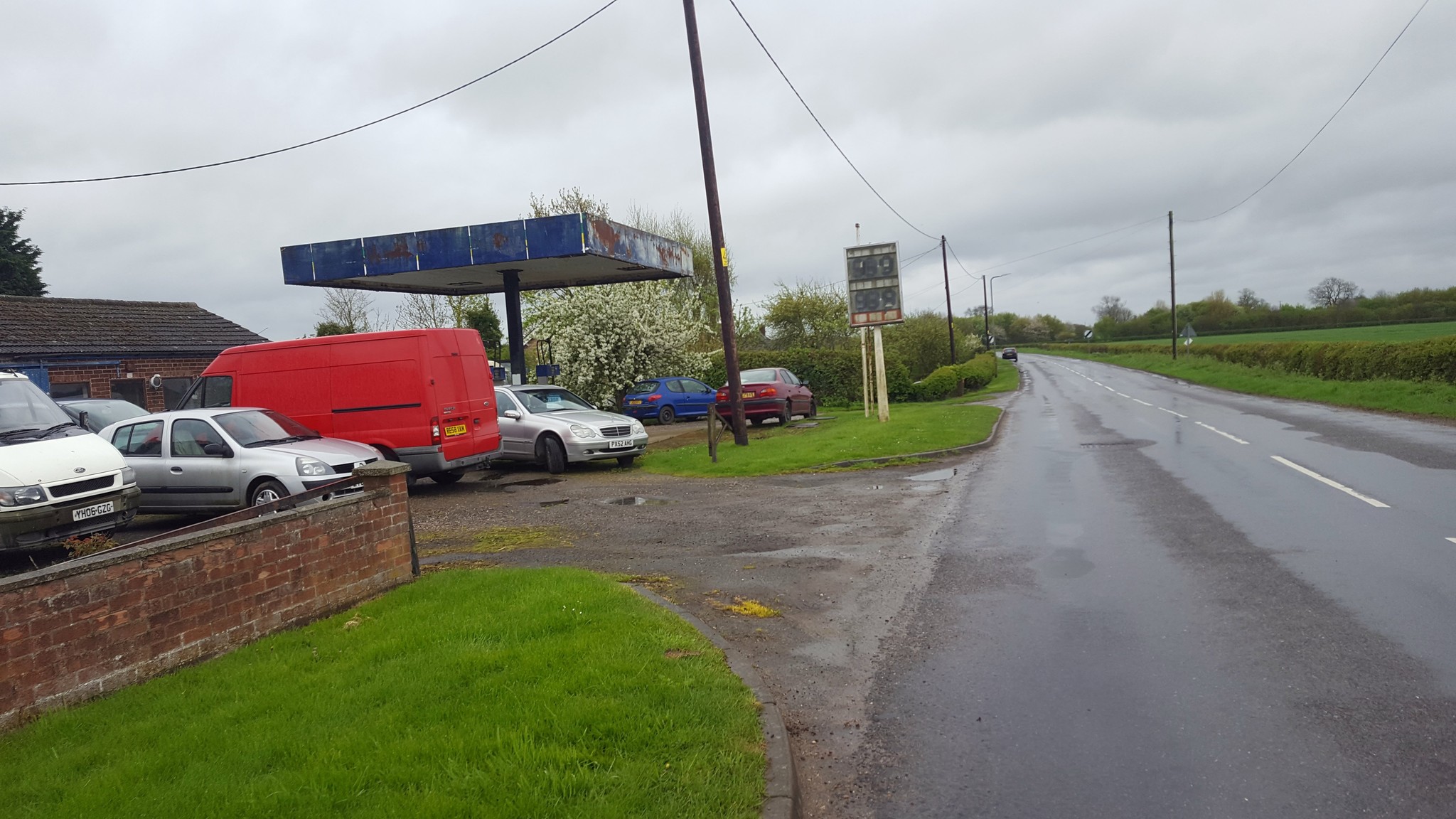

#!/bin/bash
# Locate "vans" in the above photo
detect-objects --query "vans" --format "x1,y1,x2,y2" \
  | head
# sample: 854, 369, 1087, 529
145, 327, 503, 488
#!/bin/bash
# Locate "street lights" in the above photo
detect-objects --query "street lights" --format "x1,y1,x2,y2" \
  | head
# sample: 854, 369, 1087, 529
991, 273, 1018, 377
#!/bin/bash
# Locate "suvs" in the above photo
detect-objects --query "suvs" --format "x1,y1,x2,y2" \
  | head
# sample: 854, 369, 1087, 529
0, 371, 142, 551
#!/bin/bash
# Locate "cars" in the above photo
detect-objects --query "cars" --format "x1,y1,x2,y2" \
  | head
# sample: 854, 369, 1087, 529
1002, 347, 1018, 361
716, 368, 817, 425
621, 377, 717, 425
492, 385, 649, 474
96, 407, 386, 514
0, 398, 163, 450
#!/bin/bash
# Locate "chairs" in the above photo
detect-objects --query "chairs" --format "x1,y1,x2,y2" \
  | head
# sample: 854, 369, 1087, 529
173, 430, 207, 455
